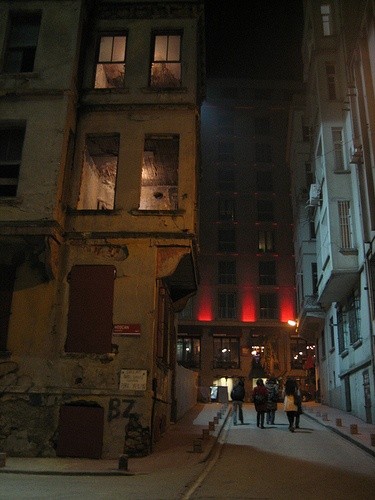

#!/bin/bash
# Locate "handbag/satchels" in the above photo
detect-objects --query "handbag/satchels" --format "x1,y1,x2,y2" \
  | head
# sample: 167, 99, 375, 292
294, 391, 298, 404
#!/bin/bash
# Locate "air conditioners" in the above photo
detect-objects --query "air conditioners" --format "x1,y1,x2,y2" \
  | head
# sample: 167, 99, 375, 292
310, 184, 320, 205
348, 143, 359, 163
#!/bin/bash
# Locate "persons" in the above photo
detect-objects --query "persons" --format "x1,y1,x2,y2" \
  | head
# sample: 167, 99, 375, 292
264, 376, 279, 423
252, 379, 269, 428
231, 380, 246, 425
282, 378, 304, 431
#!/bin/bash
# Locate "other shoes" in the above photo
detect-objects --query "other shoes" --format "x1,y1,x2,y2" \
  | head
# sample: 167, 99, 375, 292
240, 419, 243, 424
296, 426, 300, 429
289, 426, 294, 432
261, 426, 265, 429
